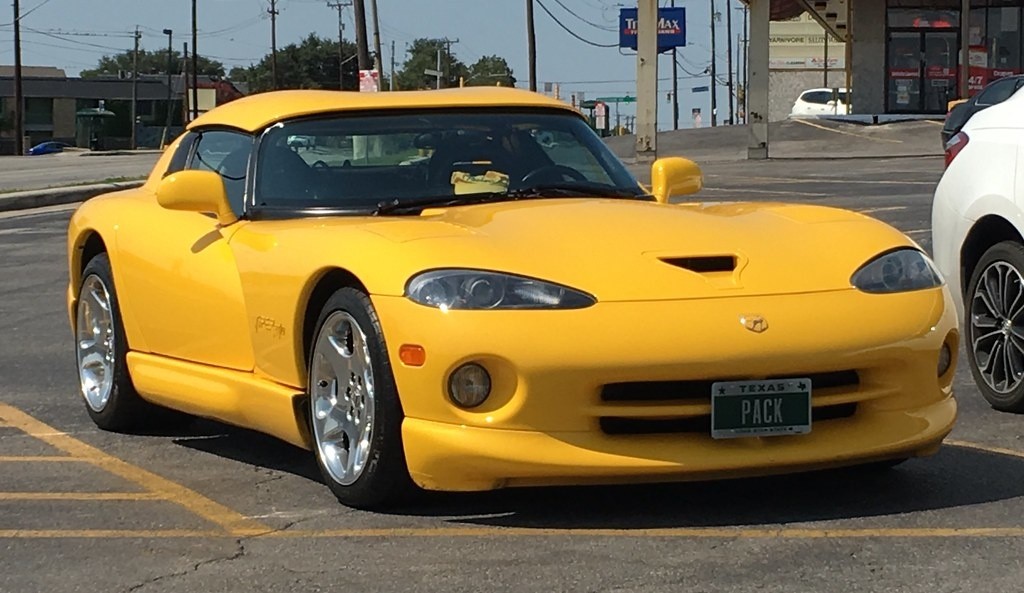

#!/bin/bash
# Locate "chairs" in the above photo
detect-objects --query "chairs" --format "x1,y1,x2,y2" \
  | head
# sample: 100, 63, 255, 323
214, 144, 317, 209
425, 134, 519, 197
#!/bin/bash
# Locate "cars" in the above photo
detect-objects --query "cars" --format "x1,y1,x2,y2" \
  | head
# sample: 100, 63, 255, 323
931, 75, 1024, 411
788, 88, 852, 115
28, 142, 72, 155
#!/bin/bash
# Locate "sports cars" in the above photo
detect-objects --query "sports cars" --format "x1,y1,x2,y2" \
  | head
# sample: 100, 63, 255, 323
68, 86, 958, 503
